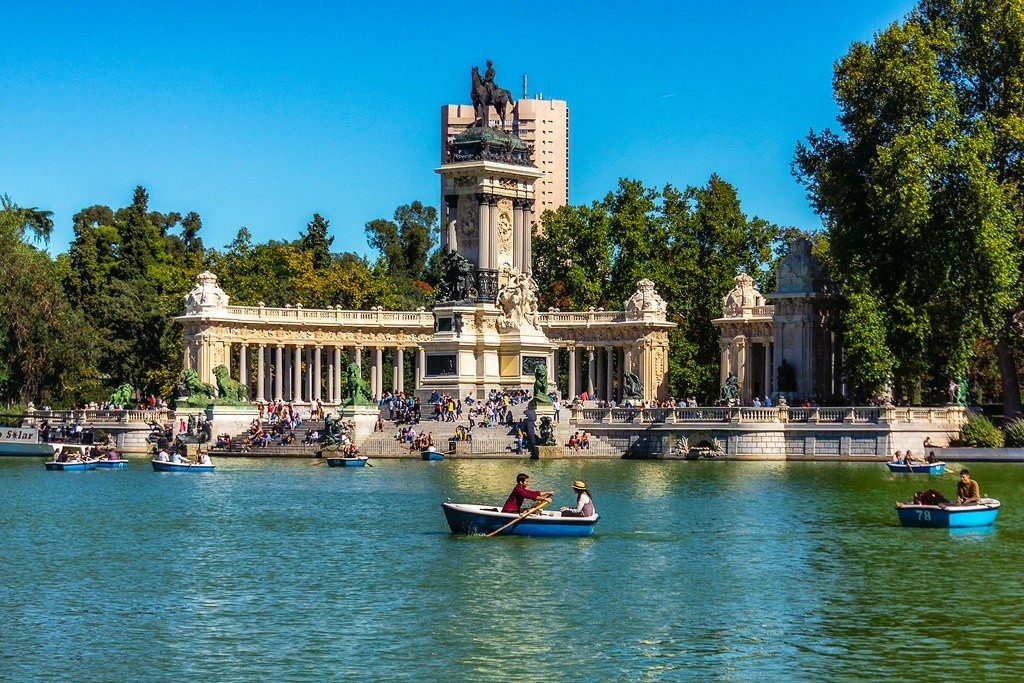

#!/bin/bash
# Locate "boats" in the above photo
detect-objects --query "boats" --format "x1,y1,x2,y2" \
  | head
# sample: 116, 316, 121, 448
326, 456, 369, 467
420, 451, 445, 460
885, 460, 946, 474
895, 497, 1002, 529
91, 458, 130, 468
440, 501, 601, 537
45, 459, 100, 471
151, 459, 216, 472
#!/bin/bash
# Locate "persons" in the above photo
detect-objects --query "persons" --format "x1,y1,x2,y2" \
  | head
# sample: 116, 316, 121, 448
722, 371, 738, 399
641, 396, 697, 416
442, 249, 464, 279
180, 412, 205, 435
560, 480, 595, 517
32, 420, 94, 444
159, 449, 211, 465
753, 395, 771, 407
625, 399, 632, 408
552, 398, 560, 423
99, 401, 123, 416
777, 358, 794, 391
924, 436, 930, 447
623, 371, 642, 397
483, 59, 496, 105
141, 394, 162, 410
501, 474, 555, 514
54, 445, 124, 463
714, 395, 740, 418
803, 398, 817, 407
580, 390, 596, 400
570, 432, 590, 450
596, 398, 616, 409
341, 422, 360, 458
956, 469, 980, 504
506, 273, 539, 328
216, 399, 323, 453
373, 387, 527, 454
892, 450, 937, 464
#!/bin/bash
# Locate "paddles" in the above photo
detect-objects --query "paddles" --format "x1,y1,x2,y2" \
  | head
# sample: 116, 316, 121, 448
485, 501, 548, 538
312, 460, 326, 466
189, 461, 193, 468
532, 493, 554, 508
444, 448, 464, 454
80, 458, 99, 470
358, 458, 374, 467
400, 445, 421, 453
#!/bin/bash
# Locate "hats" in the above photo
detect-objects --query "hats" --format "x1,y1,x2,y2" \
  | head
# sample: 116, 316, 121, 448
571, 480, 587, 490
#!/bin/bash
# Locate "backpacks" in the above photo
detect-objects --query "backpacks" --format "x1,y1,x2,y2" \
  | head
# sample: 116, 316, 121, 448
278, 403, 283, 411
